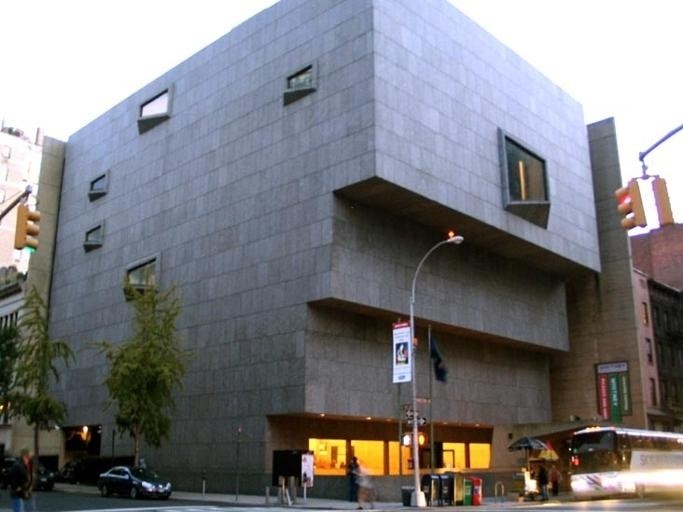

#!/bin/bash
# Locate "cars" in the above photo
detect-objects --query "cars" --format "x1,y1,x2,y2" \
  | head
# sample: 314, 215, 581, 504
5, 453, 55, 491
100, 464, 172, 499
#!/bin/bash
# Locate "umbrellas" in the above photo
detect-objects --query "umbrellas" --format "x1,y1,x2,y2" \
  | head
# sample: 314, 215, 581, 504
508, 434, 546, 468
537, 439, 559, 462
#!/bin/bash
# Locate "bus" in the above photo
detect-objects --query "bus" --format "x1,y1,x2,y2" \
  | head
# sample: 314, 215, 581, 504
567, 425, 683, 499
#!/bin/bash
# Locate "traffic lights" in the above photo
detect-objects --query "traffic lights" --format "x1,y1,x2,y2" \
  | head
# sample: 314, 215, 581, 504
613, 178, 648, 231
13, 204, 41, 251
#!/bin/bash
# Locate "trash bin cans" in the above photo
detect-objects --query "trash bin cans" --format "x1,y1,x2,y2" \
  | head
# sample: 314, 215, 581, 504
401, 486, 415, 506
421, 474, 454, 507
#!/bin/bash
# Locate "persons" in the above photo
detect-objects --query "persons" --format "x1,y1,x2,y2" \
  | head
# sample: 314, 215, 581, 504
550, 462, 564, 494
354, 461, 376, 508
538, 464, 549, 500
8, 445, 39, 511
346, 456, 360, 502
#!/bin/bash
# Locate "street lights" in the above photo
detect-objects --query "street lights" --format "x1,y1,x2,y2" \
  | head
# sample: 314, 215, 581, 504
410, 232, 465, 492
